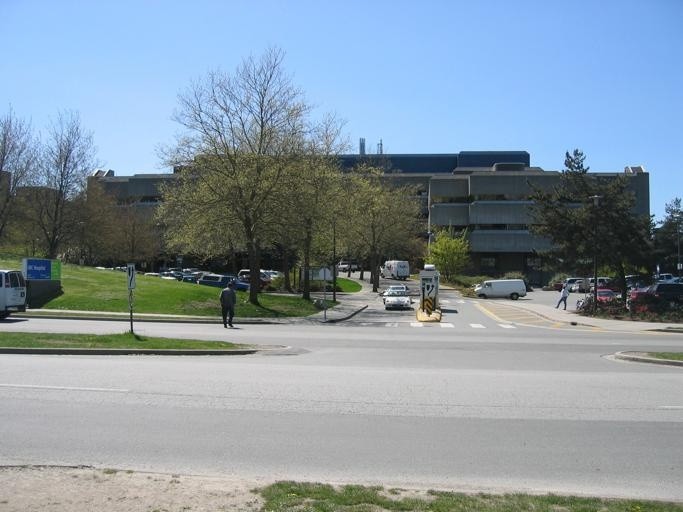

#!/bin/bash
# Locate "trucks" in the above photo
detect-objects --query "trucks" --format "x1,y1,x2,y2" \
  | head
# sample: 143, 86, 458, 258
0, 268, 29, 323
474, 279, 528, 302
379, 258, 415, 282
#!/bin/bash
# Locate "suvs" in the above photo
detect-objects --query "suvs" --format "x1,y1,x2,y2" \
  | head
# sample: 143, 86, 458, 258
335, 260, 359, 272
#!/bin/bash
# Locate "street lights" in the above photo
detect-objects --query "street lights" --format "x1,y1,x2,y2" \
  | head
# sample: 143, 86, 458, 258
671, 212, 681, 277
586, 192, 604, 317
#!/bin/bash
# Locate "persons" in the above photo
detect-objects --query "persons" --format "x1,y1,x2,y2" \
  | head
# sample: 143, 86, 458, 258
219, 282, 236, 328
555, 287, 570, 310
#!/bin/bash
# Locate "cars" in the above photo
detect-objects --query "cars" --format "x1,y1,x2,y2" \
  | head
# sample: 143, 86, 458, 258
381, 285, 414, 308
549, 272, 682, 313
96, 265, 285, 293
